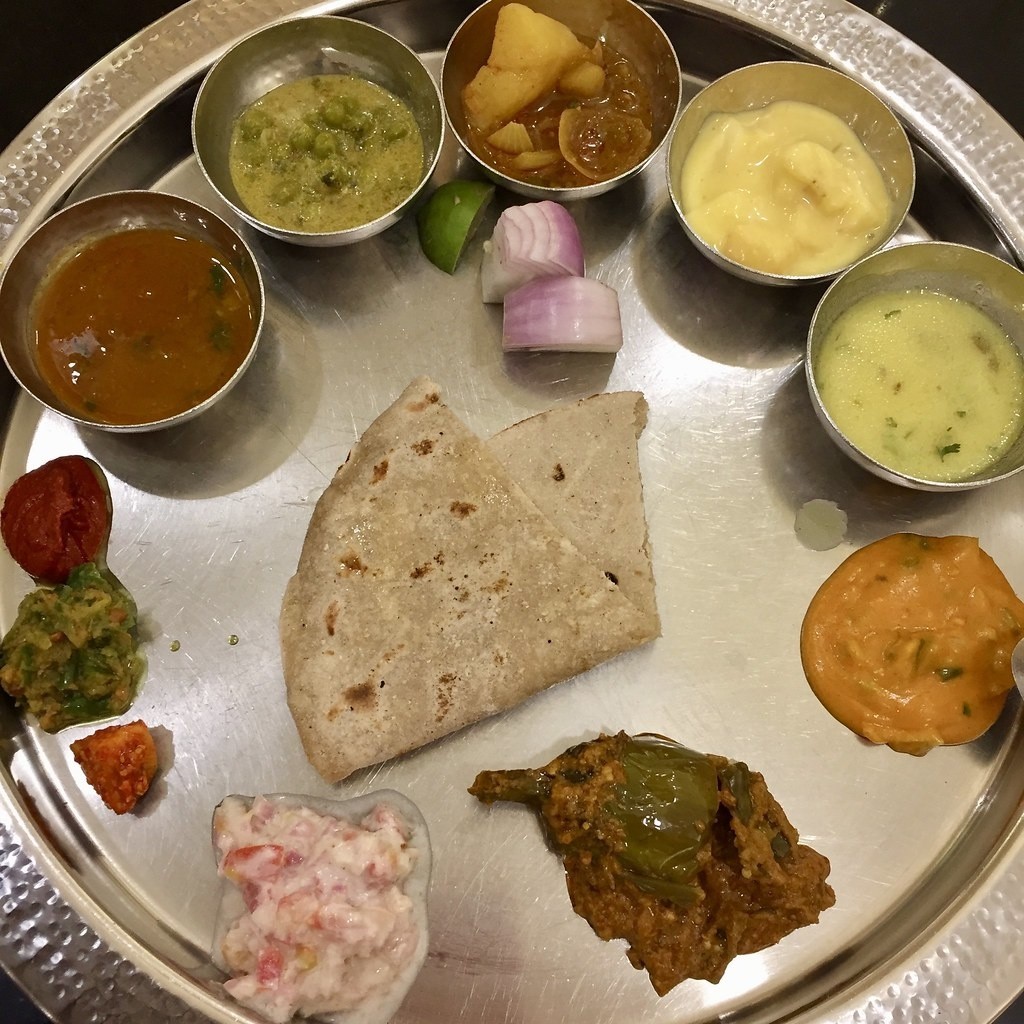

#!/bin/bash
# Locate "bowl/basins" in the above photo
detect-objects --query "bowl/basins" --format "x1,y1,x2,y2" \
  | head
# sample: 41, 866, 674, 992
0, 189, 265, 433
666, 60, 916, 287
189, 14, 445, 248
804, 241, 1024, 492
441, 0, 683, 203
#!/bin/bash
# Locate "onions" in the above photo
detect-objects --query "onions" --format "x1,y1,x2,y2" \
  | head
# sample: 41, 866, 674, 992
481, 199, 623, 353
487, 109, 652, 183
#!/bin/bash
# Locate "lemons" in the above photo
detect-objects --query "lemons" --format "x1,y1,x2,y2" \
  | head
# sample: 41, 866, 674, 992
418, 179, 495, 275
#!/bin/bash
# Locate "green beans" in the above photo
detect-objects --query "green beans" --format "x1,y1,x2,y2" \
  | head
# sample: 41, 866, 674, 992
240, 96, 409, 205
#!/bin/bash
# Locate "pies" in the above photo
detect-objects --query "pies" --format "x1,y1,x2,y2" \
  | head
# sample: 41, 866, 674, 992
278, 372, 663, 783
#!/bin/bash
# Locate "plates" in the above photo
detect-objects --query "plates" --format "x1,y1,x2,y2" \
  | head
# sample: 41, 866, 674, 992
0, 0, 1024, 1024
